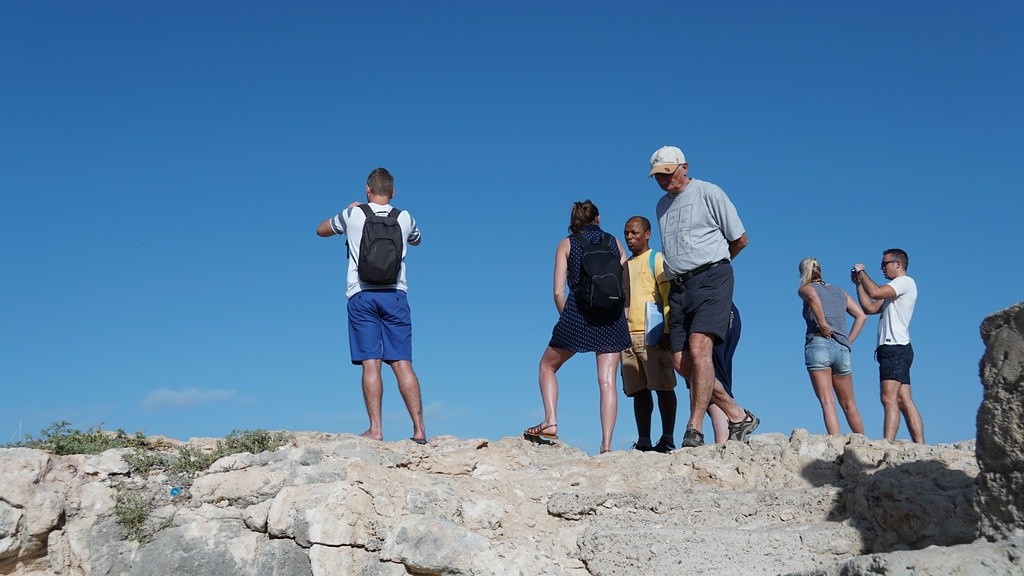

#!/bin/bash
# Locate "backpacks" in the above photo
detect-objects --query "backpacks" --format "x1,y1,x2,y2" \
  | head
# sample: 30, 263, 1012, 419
566, 232, 626, 326
346, 204, 405, 285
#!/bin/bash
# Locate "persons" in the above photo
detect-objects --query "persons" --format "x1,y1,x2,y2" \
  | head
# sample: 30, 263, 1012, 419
797, 257, 867, 436
685, 301, 741, 444
316, 167, 428, 444
648, 145, 760, 448
851, 248, 925, 444
524, 200, 630, 454
620, 216, 678, 452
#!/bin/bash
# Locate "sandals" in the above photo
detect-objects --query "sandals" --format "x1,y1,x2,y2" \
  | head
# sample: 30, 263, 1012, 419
524, 423, 558, 439
601, 450, 611, 454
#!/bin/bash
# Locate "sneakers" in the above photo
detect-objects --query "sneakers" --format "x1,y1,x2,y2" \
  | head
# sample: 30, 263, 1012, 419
682, 424, 705, 447
728, 409, 760, 441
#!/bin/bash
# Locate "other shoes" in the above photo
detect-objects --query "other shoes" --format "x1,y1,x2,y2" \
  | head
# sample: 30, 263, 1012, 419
630, 442, 653, 451
653, 439, 675, 453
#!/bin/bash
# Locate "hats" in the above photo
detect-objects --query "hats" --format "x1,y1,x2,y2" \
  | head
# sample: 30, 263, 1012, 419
647, 145, 686, 176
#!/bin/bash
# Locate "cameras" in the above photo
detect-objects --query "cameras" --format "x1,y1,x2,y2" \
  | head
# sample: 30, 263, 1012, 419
851, 268, 859, 275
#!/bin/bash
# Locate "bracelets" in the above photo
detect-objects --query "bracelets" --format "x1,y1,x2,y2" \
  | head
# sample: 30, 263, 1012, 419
857, 269, 865, 274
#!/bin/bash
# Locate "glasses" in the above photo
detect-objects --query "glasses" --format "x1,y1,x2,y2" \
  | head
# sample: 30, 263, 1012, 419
881, 260, 899, 267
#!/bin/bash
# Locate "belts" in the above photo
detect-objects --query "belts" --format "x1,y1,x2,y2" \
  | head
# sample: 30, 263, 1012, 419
670, 260, 730, 286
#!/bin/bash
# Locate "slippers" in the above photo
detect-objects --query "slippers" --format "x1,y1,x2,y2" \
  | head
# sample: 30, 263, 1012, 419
411, 438, 427, 443
